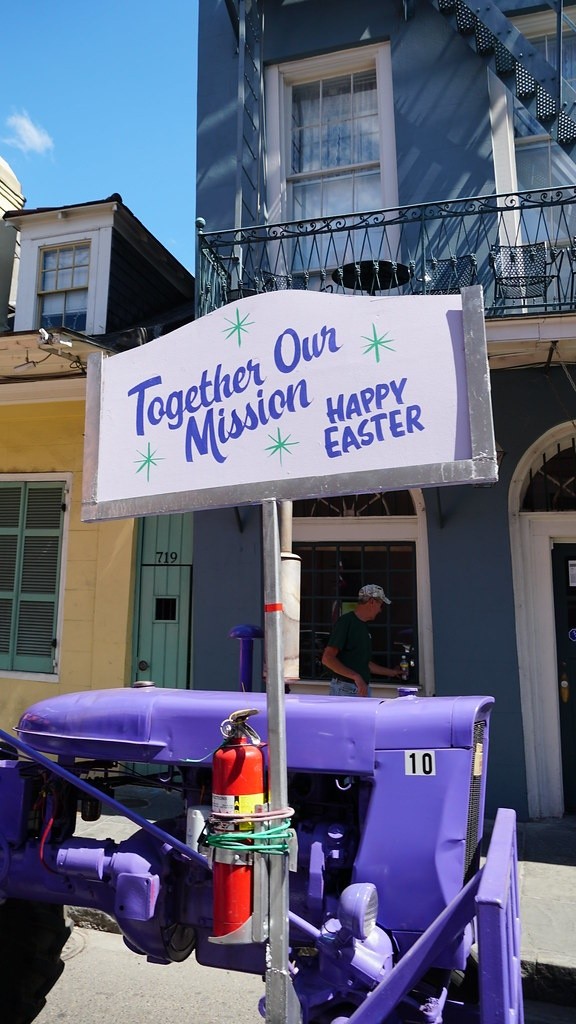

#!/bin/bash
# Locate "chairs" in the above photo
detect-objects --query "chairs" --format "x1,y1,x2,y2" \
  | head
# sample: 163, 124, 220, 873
490, 240, 557, 315
415, 255, 478, 295
254, 266, 333, 292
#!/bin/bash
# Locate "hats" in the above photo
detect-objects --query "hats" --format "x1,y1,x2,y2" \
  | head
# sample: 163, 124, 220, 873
359, 584, 392, 605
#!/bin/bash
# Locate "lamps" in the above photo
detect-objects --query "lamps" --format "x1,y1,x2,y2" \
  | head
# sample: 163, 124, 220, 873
472, 439, 508, 488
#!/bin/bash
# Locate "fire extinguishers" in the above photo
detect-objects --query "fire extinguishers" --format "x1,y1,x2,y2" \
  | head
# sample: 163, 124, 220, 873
210, 708, 269, 939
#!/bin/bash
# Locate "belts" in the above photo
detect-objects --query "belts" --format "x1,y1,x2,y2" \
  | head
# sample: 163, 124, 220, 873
332, 675, 355, 683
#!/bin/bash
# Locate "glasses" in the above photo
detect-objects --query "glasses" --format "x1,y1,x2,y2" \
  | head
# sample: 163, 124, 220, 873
369, 597, 386, 610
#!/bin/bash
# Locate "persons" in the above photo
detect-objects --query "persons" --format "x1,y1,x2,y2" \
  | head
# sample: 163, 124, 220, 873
323, 584, 406, 697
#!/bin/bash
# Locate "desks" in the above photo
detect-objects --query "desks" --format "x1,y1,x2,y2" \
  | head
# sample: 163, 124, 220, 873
331, 260, 414, 296
221, 288, 261, 302
